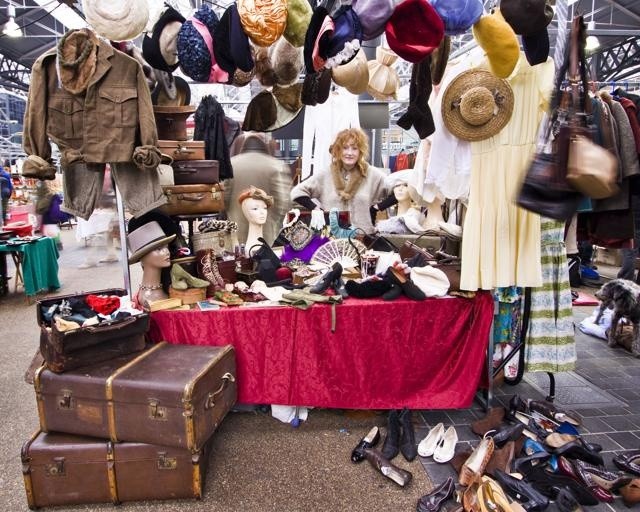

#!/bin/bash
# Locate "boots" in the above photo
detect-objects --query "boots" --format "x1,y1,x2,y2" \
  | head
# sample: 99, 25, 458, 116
450, 441, 515, 476
382, 410, 400, 461
398, 409, 417, 461
195, 248, 225, 295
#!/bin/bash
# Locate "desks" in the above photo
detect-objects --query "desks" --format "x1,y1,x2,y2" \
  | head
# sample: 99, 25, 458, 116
135, 286, 494, 415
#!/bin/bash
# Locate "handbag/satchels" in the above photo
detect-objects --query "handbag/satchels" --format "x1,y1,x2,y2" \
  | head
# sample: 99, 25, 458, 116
361, 227, 399, 253
566, 134, 619, 200
399, 231, 446, 263
249, 237, 280, 271
439, 258, 460, 292
516, 152, 583, 220
285, 220, 314, 251
198, 219, 237, 233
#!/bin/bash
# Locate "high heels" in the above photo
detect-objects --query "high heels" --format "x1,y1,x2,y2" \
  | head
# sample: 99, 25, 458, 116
170, 270, 188, 290
172, 262, 210, 287
556, 437, 603, 466
510, 394, 529, 417
574, 459, 632, 492
495, 467, 548, 512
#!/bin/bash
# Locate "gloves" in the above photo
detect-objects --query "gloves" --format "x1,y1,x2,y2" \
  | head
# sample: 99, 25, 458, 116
370, 205, 379, 225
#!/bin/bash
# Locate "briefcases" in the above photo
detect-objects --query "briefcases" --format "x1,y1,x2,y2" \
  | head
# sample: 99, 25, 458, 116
156, 182, 224, 216
36, 287, 150, 372
158, 140, 206, 160
171, 160, 220, 184
191, 230, 233, 254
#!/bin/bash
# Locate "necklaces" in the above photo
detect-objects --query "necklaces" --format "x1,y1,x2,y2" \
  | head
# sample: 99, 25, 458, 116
139, 283, 162, 290
342, 168, 354, 180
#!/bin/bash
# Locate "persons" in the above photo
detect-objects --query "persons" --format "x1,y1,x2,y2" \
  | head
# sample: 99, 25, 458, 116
386, 170, 413, 217
125, 221, 177, 310
288, 127, 399, 229
38, 179, 63, 250
240, 185, 273, 254
227, 134, 287, 255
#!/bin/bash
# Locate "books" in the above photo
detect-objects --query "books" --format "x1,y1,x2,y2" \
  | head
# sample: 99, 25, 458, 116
197, 300, 220, 316
209, 296, 227, 311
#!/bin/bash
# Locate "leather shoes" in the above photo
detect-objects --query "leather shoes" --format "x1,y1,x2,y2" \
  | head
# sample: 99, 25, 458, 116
529, 401, 585, 426
524, 439, 559, 474
482, 476, 527, 512
351, 426, 380, 462
588, 443, 602, 453
333, 277, 349, 299
433, 425, 458, 464
515, 452, 552, 478
613, 452, 640, 476
477, 480, 502, 512
386, 267, 425, 301
417, 477, 454, 512
463, 474, 483, 512
310, 262, 342, 293
619, 479, 640, 507
363, 449, 412, 488
507, 411, 549, 440
558, 456, 615, 504
458, 436, 495, 487
418, 423, 445, 457
382, 284, 401, 300
493, 423, 523, 448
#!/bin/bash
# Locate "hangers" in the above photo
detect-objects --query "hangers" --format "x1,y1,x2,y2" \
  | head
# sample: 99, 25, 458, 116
584, 79, 640, 104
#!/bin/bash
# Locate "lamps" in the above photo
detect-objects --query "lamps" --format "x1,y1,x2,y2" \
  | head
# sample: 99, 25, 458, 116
583, 0, 606, 54
2, 5, 25, 42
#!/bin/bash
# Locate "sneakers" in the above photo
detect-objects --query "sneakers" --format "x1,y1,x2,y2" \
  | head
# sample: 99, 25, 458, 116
546, 432, 577, 447
472, 408, 505, 435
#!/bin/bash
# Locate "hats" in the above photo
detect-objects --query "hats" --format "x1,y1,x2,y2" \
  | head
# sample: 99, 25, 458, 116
268, 38, 304, 85
82, 0, 149, 41
351, 0, 396, 41
441, 68, 514, 141
472, 6, 520, 78
213, 5, 256, 86
282, 0, 314, 46
177, 3, 229, 83
143, 3, 187, 72
324, 8, 362, 69
385, 0, 445, 63
431, 0, 483, 34
151, 75, 191, 106
127, 220, 177, 265
332, 47, 369, 94
304, 6, 335, 74
237, 0, 287, 47
501, 0, 553, 34
